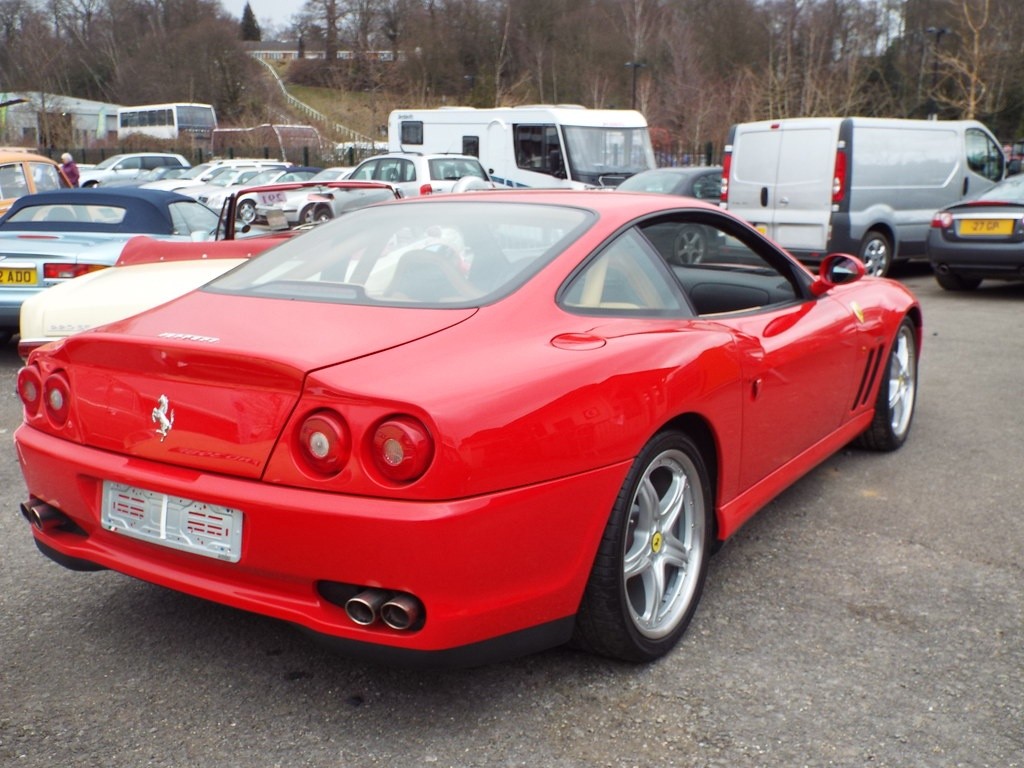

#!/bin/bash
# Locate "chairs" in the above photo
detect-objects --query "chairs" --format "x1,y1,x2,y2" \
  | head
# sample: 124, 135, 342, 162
443, 164, 457, 179
381, 248, 473, 303
387, 167, 398, 183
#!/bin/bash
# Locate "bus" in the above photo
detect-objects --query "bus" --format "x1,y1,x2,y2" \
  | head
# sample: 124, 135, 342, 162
327, 140, 390, 167
114, 102, 220, 153
209, 123, 324, 169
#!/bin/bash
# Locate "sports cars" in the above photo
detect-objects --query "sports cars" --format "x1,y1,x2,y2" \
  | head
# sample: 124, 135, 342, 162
18, 221, 476, 371
12, 182, 925, 667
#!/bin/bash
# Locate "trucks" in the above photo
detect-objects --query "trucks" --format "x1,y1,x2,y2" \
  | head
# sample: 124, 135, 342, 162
385, 100, 661, 193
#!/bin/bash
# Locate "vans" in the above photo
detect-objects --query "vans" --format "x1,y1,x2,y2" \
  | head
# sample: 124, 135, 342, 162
716, 116, 1023, 278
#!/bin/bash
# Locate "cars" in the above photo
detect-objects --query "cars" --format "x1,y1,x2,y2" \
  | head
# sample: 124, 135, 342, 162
612, 161, 724, 268
924, 171, 1023, 293
0, 136, 501, 238
0, 186, 269, 331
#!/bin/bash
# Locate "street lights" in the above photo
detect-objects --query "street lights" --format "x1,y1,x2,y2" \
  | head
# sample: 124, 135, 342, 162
925, 25, 954, 119
624, 60, 647, 109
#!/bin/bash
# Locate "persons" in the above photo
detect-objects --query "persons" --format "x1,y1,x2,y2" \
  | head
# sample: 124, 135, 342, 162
59, 153, 80, 189
